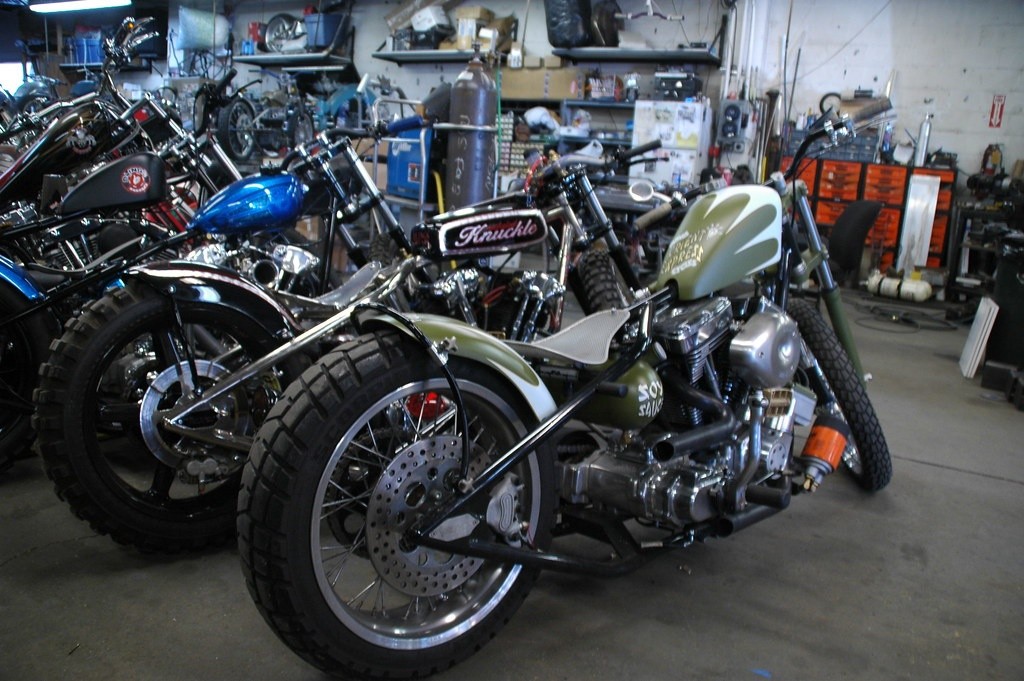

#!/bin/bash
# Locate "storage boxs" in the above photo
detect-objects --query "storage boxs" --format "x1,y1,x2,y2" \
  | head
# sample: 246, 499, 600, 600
409, 5, 449, 32
68, 37, 103, 64
305, 13, 342, 46
386, 116, 433, 200
789, 127, 877, 161
626, 98, 714, 189
438, 5, 513, 50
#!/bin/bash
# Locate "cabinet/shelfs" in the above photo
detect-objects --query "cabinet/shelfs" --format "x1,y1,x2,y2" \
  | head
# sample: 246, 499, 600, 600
777, 155, 958, 275
557, 96, 635, 184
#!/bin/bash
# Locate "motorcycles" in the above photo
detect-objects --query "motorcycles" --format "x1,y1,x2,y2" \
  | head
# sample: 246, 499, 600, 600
0, 16, 670, 557
236, 98, 893, 681
